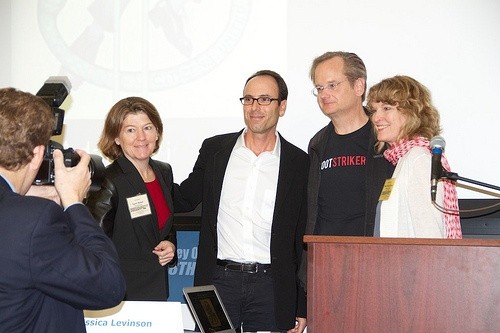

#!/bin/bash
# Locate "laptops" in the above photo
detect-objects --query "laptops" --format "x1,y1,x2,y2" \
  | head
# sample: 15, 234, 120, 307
182, 286, 240, 333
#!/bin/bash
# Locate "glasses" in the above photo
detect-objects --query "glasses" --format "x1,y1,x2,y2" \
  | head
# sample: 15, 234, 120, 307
240, 95, 280, 106
312, 80, 346, 96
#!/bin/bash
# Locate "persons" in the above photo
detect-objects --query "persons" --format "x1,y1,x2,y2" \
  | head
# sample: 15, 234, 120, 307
0, 86, 128, 333
87, 96, 178, 302
296, 48, 396, 293
171, 68, 309, 332
366, 74, 465, 239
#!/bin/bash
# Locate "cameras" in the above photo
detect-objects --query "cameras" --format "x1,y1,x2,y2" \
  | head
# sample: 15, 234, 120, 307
30, 82, 106, 187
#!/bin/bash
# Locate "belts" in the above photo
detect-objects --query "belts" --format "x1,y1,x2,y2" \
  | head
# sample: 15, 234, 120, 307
216, 257, 270, 275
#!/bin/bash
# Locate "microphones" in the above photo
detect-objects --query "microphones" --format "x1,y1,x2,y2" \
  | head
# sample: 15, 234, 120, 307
429, 136, 445, 201
